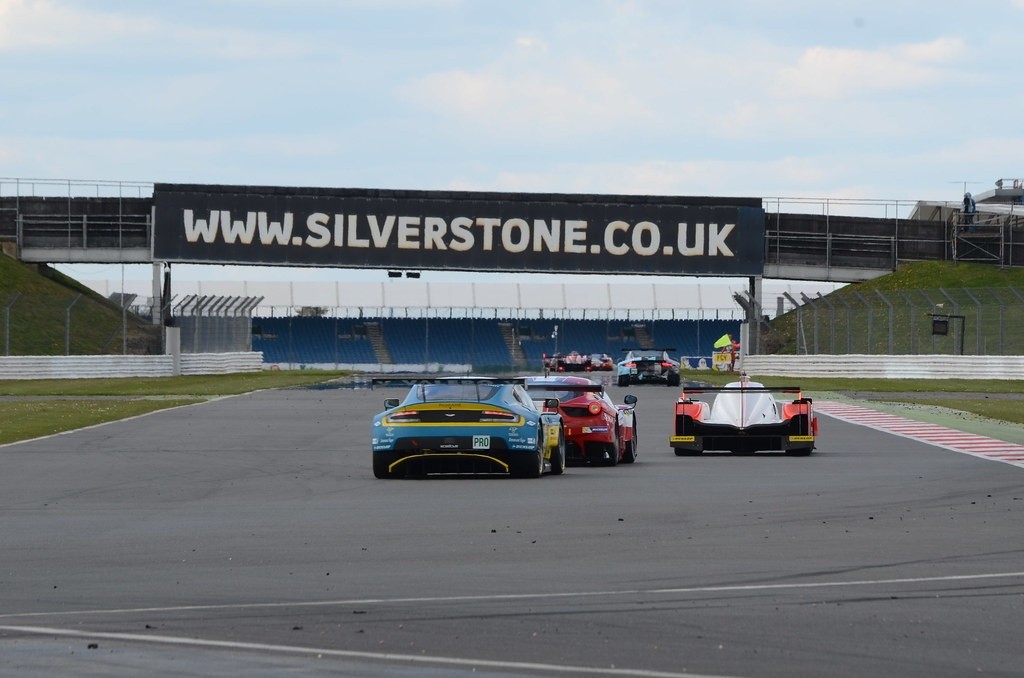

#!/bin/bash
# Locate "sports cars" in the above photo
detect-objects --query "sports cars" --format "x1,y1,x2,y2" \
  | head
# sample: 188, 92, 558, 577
368, 379, 564, 481
617, 349, 683, 388
506, 374, 641, 467
582, 353, 615, 372
669, 370, 819, 457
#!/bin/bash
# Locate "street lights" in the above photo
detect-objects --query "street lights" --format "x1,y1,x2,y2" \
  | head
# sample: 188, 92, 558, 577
551, 325, 559, 372
